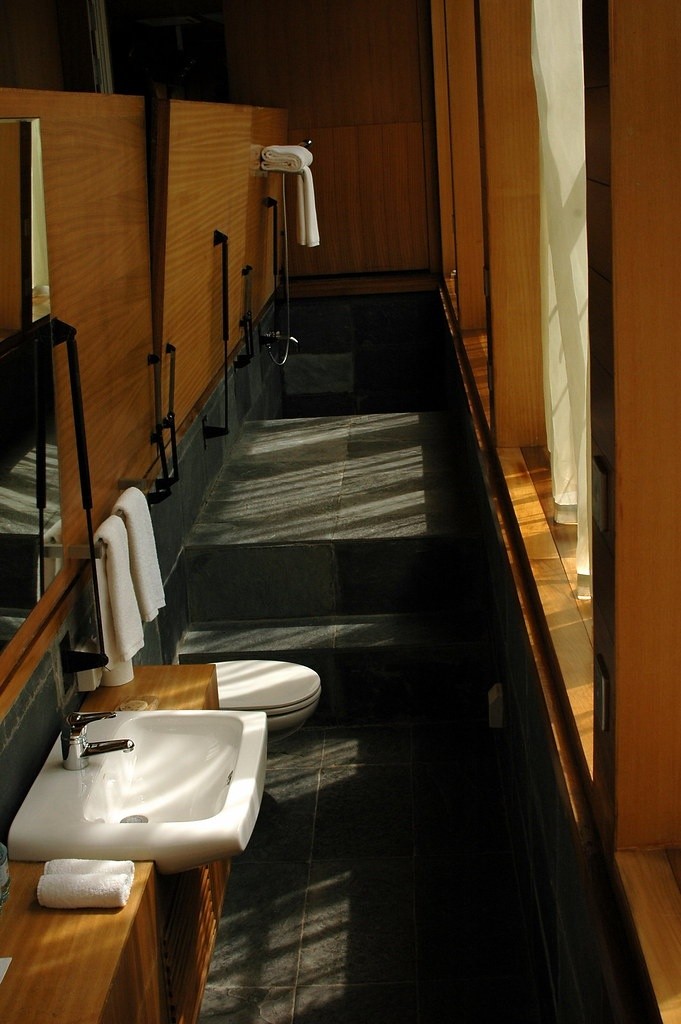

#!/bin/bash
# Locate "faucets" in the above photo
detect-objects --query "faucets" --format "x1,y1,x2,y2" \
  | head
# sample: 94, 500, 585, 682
61, 711, 135, 771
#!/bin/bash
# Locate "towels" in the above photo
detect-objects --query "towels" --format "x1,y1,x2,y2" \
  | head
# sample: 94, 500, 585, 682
43, 858, 135, 883
37, 875, 132, 908
297, 165, 321, 248
260, 144, 313, 172
93, 515, 144, 671
110, 487, 167, 623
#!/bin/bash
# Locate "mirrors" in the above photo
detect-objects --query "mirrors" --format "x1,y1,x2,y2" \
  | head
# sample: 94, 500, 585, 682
0, 117, 63, 664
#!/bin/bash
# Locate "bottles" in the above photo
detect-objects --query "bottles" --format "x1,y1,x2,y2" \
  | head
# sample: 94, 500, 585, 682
0, 842, 11, 903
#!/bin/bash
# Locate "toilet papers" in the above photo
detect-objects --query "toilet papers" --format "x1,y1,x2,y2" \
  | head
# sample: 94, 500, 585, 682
99, 657, 135, 686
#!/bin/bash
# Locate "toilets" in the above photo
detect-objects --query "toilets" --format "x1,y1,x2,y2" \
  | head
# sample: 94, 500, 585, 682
202, 660, 321, 744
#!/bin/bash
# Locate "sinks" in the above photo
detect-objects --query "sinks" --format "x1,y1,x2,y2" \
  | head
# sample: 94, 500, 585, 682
83, 715, 244, 824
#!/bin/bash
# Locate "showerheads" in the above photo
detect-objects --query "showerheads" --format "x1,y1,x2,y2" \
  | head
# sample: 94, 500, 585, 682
298, 137, 315, 150
